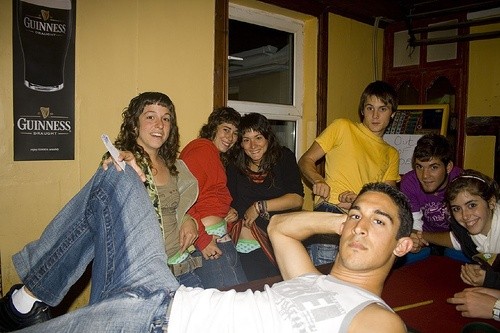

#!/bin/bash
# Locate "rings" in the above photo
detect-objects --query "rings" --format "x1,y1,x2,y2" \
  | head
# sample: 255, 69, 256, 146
207, 254, 213, 258
212, 252, 217, 257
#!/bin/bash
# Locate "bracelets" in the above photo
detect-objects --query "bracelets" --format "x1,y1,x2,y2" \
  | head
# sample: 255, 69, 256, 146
492, 299, 500, 322
253, 199, 270, 222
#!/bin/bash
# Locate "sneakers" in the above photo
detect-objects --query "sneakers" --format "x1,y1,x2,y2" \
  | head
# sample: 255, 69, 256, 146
0, 284, 50, 333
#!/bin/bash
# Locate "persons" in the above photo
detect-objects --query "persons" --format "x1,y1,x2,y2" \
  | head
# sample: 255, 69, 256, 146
423, 169, 500, 325
96, 91, 205, 291
0, 158, 414, 333
222, 113, 305, 282
297, 81, 401, 213
179, 106, 249, 289
400, 134, 462, 235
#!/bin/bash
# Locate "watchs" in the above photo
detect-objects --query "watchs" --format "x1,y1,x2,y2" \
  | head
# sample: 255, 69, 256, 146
481, 253, 493, 262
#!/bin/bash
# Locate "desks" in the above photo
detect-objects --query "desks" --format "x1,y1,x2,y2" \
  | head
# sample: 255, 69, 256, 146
217, 252, 500, 333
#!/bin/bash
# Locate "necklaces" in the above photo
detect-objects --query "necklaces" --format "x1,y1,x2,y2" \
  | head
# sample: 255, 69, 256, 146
252, 160, 261, 167
